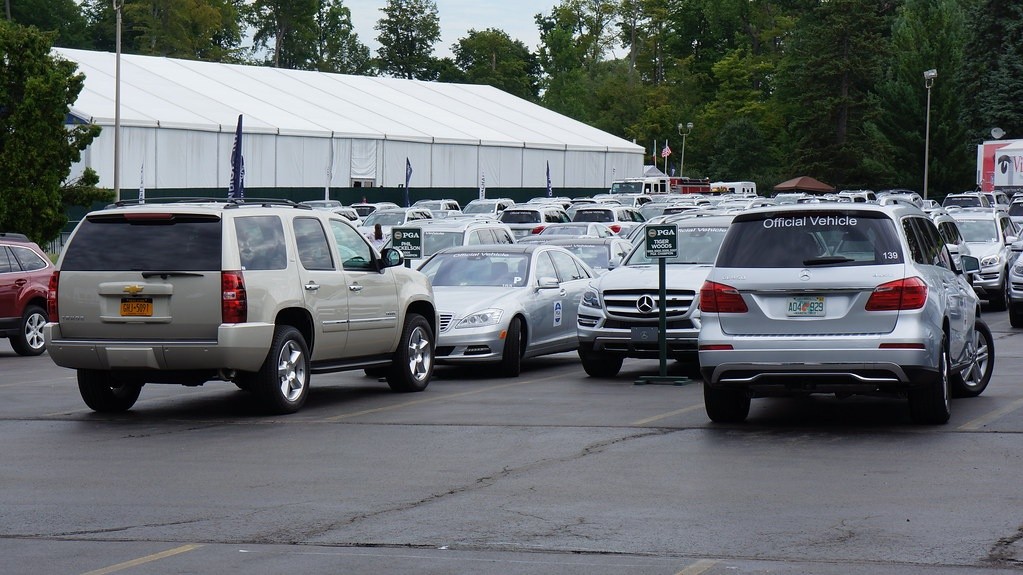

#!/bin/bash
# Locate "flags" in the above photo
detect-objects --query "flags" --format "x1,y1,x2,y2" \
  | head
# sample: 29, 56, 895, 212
227, 114, 245, 202
653, 148, 655, 161
662, 145, 671, 157
404, 158, 412, 207
480, 169, 485, 199
547, 165, 552, 198
670, 163, 675, 177
139, 158, 145, 205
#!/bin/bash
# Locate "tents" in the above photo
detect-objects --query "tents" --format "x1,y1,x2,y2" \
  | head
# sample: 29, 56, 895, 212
773, 176, 836, 193
646, 166, 664, 176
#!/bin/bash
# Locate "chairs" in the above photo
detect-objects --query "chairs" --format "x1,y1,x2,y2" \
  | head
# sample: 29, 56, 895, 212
488, 262, 508, 280
873, 234, 898, 261
516, 259, 530, 280
755, 230, 816, 263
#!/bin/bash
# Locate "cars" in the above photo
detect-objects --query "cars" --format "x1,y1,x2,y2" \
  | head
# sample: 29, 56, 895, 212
296, 175, 1023, 333
415, 243, 599, 378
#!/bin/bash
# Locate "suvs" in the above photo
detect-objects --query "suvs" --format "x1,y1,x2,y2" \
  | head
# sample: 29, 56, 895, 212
43, 197, 442, 415
0, 232, 55, 357
697, 195, 995, 424
577, 213, 830, 380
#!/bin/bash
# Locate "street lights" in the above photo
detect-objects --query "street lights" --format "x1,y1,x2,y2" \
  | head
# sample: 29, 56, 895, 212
678, 121, 693, 177
924, 68, 938, 200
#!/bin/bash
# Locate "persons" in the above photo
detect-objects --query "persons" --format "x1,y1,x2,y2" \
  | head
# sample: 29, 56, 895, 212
366, 224, 386, 249
362, 196, 366, 203
